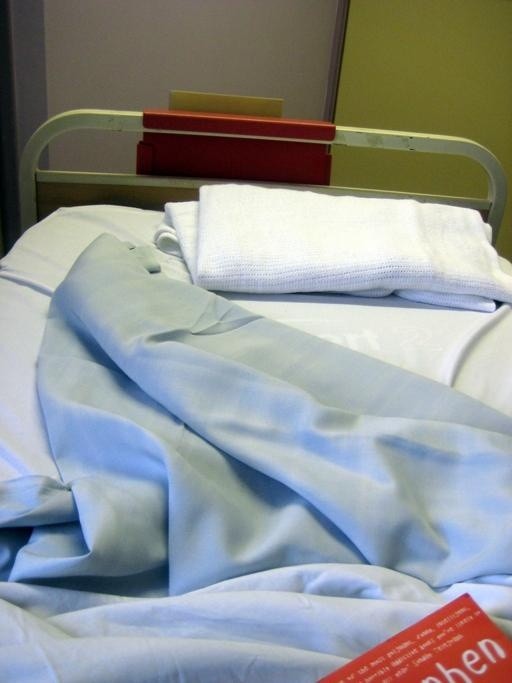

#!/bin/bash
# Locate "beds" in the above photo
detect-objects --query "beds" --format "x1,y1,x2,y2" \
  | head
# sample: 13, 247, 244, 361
0, 109, 512, 683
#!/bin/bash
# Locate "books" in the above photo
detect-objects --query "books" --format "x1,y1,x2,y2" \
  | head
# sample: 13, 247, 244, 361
315, 592, 512, 683
